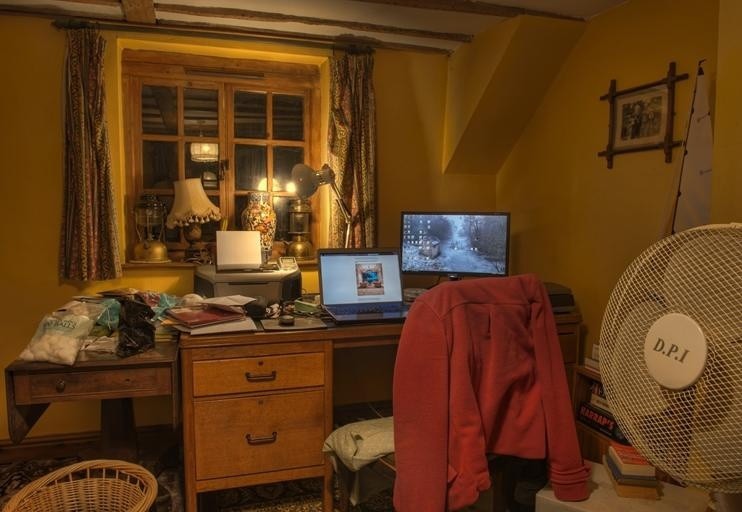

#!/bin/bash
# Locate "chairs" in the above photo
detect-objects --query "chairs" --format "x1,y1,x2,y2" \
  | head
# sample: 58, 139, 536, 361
333, 273, 551, 511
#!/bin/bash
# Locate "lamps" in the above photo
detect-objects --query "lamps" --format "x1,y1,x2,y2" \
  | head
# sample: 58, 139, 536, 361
292, 164, 353, 304
166, 177, 222, 261
189, 121, 219, 164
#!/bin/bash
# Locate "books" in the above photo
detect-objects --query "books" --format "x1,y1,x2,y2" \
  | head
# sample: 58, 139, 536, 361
164, 305, 247, 328
575, 342, 661, 500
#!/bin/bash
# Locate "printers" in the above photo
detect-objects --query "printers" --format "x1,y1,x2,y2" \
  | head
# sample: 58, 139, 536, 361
194, 230, 301, 306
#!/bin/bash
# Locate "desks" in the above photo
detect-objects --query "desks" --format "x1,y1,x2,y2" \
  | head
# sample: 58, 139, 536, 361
178, 296, 584, 512
6, 334, 181, 482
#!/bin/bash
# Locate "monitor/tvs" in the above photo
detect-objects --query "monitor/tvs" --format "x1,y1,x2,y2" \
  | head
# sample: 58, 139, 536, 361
400, 211, 511, 281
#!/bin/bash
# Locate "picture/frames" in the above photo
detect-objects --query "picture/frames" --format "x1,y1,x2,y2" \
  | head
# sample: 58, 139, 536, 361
614, 88, 668, 148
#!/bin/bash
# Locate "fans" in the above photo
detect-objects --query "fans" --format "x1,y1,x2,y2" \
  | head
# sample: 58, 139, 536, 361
597, 222, 741, 511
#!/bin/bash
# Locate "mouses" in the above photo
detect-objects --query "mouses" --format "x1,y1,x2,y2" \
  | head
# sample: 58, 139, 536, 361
280, 315, 294, 325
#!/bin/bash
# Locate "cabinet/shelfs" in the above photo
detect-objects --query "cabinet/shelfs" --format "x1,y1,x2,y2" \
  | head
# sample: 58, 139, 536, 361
569, 360, 693, 487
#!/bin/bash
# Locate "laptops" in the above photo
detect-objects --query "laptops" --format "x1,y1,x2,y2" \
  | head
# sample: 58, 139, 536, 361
318, 248, 411, 325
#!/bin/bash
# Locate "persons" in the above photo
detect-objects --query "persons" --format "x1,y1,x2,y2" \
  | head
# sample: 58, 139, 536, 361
628, 104, 661, 138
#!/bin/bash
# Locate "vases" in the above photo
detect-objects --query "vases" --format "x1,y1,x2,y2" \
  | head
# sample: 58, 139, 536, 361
241, 192, 277, 264
